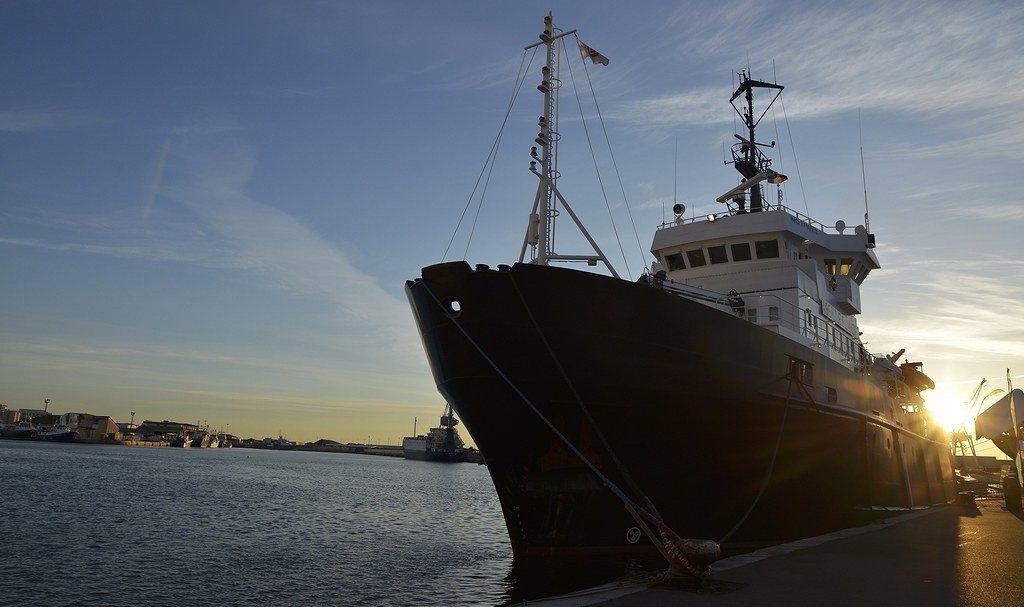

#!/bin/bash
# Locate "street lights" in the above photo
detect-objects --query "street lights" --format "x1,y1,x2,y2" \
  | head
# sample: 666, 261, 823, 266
129, 412, 135, 435
45, 399, 50, 415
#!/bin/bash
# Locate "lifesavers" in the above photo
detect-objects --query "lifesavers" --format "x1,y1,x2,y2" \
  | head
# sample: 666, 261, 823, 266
899, 365, 935, 389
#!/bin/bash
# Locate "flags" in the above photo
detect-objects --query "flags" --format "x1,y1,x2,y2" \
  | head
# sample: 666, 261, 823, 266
577, 38, 609, 66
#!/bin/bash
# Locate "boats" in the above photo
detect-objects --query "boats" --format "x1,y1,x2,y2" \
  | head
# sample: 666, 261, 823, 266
403, 403, 473, 462
161, 425, 232, 448
405, 11, 959, 578
0, 415, 77, 442
